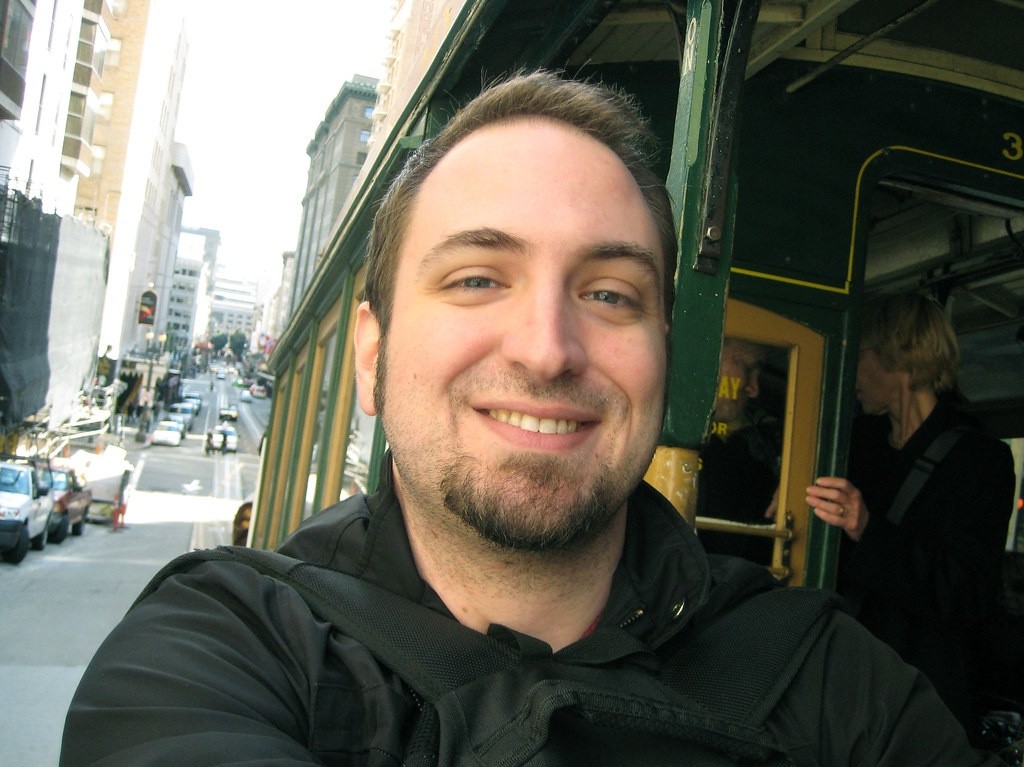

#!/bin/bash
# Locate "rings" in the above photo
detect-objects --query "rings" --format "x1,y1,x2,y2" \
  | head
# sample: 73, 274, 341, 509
839, 506, 846, 518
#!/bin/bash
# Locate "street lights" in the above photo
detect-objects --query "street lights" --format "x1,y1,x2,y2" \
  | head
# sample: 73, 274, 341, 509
134, 328, 168, 442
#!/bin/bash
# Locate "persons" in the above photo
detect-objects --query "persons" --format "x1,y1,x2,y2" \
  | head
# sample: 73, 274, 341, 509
219, 430, 227, 456
694, 337, 778, 568
59, 70, 976, 767
205, 428, 215, 455
764, 286, 1015, 747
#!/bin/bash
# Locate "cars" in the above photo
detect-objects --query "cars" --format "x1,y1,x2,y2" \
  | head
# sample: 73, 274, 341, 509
0, 450, 134, 564
240, 384, 269, 402
150, 392, 203, 446
208, 401, 239, 454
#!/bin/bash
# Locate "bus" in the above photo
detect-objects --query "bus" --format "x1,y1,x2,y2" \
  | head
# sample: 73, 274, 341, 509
246, 1, 1024, 654
